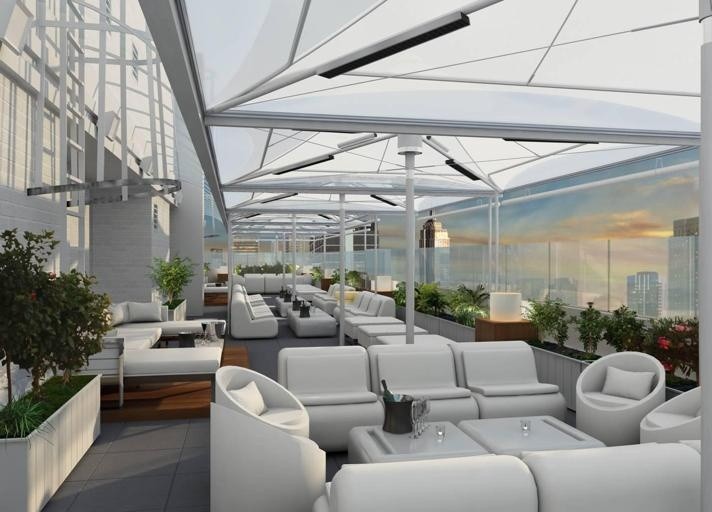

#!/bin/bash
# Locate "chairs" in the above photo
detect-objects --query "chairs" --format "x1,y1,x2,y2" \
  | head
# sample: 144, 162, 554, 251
204, 273, 396, 339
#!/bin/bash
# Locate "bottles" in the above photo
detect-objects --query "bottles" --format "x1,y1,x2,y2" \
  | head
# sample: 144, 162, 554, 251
294, 295, 298, 301
285, 289, 288, 294
280, 287, 283, 291
381, 379, 395, 402
301, 300, 306, 307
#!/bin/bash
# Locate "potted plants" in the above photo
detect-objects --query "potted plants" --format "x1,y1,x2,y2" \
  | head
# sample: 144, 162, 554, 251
144, 254, 198, 321
0, 226, 114, 512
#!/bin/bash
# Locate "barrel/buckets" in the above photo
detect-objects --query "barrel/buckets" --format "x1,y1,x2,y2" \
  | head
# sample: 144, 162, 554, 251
380, 393, 413, 434
201, 321, 224, 339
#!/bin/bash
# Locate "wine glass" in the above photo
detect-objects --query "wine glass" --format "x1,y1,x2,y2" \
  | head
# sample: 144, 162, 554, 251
408, 396, 431, 439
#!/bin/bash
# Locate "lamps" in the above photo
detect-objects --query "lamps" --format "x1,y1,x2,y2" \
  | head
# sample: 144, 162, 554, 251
96, 111, 120, 142
140, 156, 156, 176
0, 0, 34, 55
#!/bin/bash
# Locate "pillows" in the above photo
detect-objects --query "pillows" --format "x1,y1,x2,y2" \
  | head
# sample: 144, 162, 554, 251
127, 301, 163, 322
111, 301, 130, 326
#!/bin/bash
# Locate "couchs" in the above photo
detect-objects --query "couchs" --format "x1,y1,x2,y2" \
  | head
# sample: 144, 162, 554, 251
70, 306, 226, 408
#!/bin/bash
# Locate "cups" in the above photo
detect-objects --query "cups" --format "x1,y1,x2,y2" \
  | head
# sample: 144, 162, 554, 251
519, 419, 532, 437
432, 424, 446, 443
